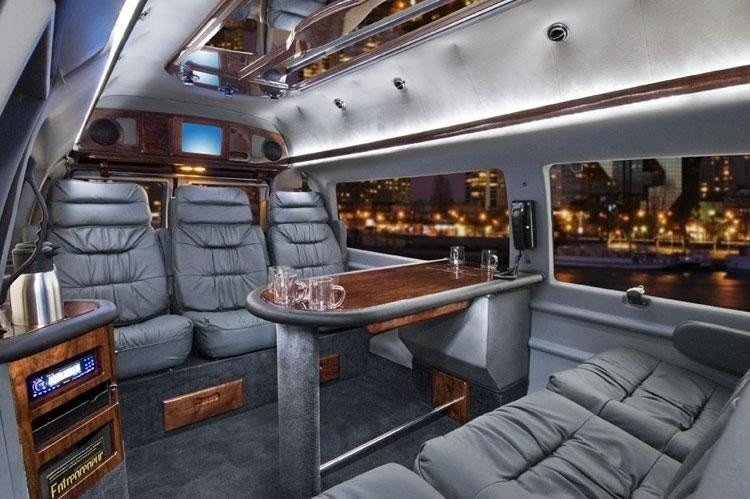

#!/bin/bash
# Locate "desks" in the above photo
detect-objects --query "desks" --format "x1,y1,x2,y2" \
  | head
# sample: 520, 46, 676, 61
244, 255, 546, 497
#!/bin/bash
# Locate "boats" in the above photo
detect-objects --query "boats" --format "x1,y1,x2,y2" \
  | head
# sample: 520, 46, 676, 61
723, 244, 750, 275
554, 241, 682, 270
679, 246, 715, 272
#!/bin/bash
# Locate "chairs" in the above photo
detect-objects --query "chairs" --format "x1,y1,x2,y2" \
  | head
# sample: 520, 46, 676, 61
544, 320, 749, 464
167, 180, 284, 362
412, 386, 750, 499
310, 460, 443, 499
45, 178, 196, 377
263, 190, 356, 336
264, 1, 333, 33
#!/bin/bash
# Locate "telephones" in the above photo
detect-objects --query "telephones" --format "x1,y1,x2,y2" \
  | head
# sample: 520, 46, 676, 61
510, 199, 536, 251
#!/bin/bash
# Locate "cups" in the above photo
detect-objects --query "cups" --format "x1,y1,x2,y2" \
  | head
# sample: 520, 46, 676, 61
450, 267, 465, 279
449, 246, 466, 266
480, 270, 500, 284
267, 265, 291, 295
480, 248, 500, 270
308, 276, 345, 311
273, 272, 305, 306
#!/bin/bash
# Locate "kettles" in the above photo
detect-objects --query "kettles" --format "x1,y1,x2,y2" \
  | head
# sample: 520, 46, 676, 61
10, 240, 67, 326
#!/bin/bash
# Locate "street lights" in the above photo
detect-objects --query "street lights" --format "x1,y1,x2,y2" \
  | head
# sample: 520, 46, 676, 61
557, 209, 741, 255
353, 209, 509, 237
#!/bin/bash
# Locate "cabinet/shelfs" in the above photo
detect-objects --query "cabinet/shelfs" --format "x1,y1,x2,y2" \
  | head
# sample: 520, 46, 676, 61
0, 293, 133, 499
